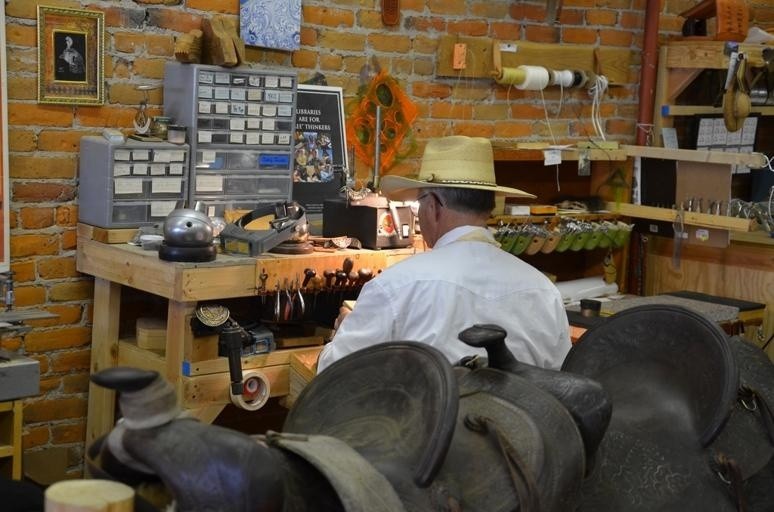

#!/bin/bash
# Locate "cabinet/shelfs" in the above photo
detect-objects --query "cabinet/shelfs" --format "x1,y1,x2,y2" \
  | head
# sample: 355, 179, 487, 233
489, 142, 634, 310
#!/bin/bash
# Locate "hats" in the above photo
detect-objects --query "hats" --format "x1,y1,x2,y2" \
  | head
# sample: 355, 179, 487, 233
723, 77, 752, 133
379, 134, 539, 201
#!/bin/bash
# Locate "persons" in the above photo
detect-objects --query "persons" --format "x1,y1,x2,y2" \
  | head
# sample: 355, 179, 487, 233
316, 135, 572, 376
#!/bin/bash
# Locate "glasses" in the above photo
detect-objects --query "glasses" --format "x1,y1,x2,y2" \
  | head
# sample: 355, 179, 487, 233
409, 192, 444, 218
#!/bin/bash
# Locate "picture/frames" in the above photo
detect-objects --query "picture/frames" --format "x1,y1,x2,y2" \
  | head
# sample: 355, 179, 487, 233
35, 3, 106, 109
279, 80, 350, 223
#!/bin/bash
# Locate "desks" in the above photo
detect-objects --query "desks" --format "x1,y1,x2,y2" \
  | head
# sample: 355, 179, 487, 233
75, 236, 426, 477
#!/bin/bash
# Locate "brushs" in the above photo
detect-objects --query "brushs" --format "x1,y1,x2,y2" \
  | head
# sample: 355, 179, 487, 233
731, 58, 752, 119
